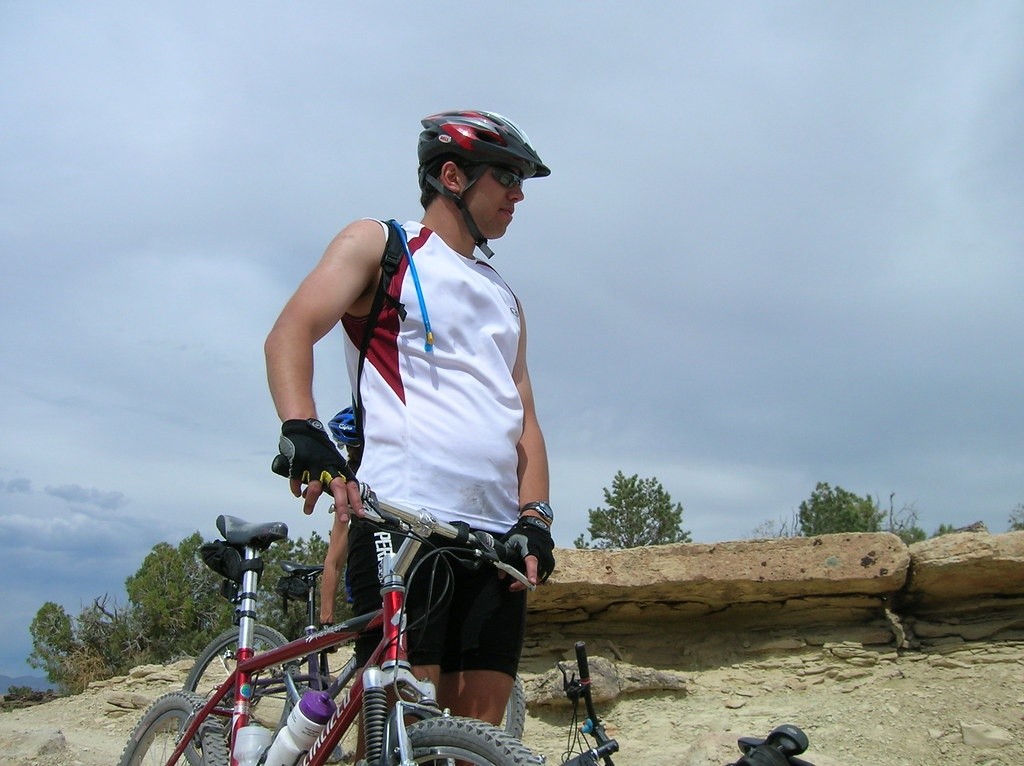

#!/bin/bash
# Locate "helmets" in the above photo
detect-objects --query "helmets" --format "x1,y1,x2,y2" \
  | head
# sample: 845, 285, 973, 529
418, 109, 551, 178
326, 407, 364, 448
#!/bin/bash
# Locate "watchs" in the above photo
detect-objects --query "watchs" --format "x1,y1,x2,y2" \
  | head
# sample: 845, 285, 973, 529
520, 501, 554, 524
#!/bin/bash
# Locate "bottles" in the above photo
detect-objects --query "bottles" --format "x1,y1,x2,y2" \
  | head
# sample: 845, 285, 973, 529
232, 721, 273, 766
264, 690, 336, 766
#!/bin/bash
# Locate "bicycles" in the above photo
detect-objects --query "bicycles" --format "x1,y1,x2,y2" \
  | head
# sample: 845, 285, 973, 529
116, 450, 557, 766
551, 638, 834, 766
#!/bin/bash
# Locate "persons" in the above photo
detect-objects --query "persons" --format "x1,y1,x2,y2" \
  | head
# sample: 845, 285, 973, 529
263, 107, 555, 766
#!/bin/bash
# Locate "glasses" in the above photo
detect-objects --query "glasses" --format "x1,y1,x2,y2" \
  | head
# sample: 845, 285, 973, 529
491, 167, 524, 190
